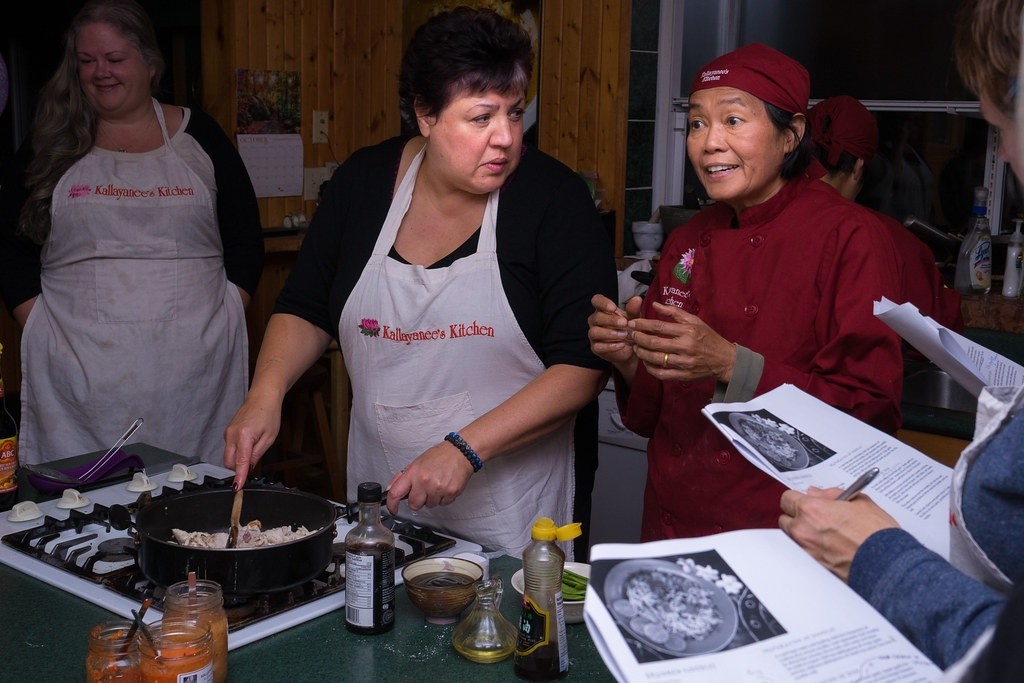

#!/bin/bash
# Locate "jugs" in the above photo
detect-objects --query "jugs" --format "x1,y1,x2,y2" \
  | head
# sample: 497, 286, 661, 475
452, 578, 518, 663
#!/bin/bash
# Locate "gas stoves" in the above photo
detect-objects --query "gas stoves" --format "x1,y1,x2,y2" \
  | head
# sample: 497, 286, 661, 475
0, 463, 482, 652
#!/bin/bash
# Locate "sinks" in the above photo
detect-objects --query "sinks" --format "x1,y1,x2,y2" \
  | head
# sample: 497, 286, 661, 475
934, 261, 1004, 290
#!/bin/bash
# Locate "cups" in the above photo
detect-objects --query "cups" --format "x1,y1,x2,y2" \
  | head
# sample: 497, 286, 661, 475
451, 550, 489, 581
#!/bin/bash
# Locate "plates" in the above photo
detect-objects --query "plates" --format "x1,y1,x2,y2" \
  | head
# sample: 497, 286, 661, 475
728, 412, 809, 470
796, 427, 837, 461
737, 585, 787, 641
603, 558, 739, 657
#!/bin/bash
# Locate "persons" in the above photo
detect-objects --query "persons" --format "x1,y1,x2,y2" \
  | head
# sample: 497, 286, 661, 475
0, 0, 263, 470
588, 43, 948, 542
779, 0, 1024, 683
873, 108, 938, 248
223, 5, 621, 563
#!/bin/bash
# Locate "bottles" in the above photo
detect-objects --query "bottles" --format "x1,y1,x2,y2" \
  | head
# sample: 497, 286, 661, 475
0, 344, 17, 512
86, 579, 228, 683
345, 482, 396, 636
513, 517, 582, 683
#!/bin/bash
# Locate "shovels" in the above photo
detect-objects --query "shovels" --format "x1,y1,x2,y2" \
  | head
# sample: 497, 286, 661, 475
225, 489, 243, 549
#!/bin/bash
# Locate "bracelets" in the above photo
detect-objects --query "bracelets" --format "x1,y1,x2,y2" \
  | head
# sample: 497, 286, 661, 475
448, 432, 482, 469
444, 436, 477, 473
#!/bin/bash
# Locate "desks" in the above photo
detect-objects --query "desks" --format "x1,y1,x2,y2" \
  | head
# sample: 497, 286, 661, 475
248, 230, 306, 361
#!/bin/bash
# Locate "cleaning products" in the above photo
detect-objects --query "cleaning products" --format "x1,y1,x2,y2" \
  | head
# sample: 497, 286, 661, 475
954, 185, 994, 295
1000, 218, 1024, 299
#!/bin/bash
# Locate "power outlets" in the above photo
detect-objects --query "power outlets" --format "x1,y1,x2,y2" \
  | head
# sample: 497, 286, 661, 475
313, 110, 328, 144
304, 168, 327, 201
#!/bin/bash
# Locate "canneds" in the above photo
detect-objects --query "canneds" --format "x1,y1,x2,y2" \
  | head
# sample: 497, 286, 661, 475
85, 579, 229, 683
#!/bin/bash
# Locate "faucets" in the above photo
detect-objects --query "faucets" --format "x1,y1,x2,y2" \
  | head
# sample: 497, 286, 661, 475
903, 213, 954, 242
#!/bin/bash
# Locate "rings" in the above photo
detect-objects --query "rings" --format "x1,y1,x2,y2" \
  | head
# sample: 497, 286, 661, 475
663, 353, 669, 368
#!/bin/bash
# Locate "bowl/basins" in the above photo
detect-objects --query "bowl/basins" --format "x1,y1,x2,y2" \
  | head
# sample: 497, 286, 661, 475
510, 562, 592, 623
401, 558, 483, 625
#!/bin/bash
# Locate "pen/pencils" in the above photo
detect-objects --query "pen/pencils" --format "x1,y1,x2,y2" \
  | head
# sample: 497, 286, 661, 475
835, 466, 880, 502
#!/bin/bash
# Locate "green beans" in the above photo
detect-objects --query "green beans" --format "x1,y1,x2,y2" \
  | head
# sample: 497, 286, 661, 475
561, 569, 588, 602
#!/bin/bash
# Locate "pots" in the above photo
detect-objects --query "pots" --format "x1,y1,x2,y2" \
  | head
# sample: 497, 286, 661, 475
134, 488, 409, 595
902, 213, 1024, 276
